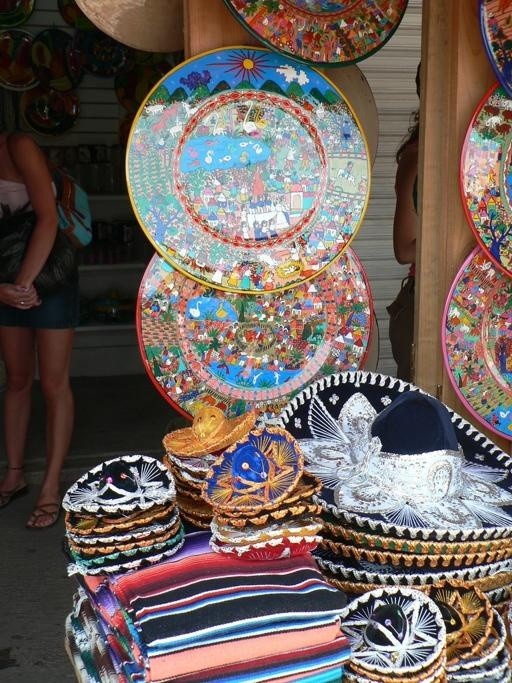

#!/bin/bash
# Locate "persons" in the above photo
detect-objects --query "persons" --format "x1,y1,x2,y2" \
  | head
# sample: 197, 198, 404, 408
0, 106, 80, 531
384, 64, 421, 381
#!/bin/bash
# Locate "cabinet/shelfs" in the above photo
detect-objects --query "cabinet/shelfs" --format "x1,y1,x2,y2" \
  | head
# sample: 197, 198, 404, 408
72, 187, 149, 332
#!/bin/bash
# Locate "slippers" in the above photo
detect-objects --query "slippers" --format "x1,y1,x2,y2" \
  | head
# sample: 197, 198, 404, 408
25, 497, 61, 530
0, 481, 27, 507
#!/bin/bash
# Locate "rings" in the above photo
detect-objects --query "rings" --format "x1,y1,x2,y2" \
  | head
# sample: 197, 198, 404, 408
19, 300, 25, 306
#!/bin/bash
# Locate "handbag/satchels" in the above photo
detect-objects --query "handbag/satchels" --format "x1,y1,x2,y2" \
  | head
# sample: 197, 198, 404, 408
51, 167, 94, 248
